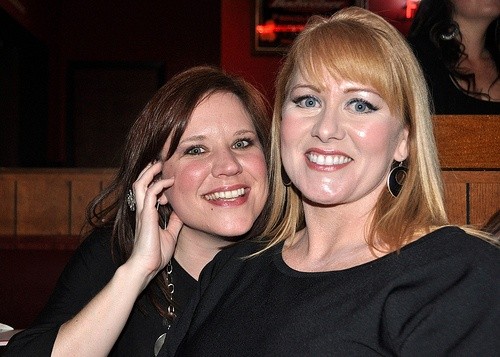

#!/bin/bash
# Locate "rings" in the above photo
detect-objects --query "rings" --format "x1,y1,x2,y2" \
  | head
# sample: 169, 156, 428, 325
126, 190, 136, 211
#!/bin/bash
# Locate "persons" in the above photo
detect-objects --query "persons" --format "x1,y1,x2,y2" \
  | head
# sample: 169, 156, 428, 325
156, 7, 500, 357
0, 65, 302, 357
405, 0, 499, 114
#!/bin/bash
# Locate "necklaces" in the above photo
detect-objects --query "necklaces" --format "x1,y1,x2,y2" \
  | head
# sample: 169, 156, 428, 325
154, 256, 178, 357
